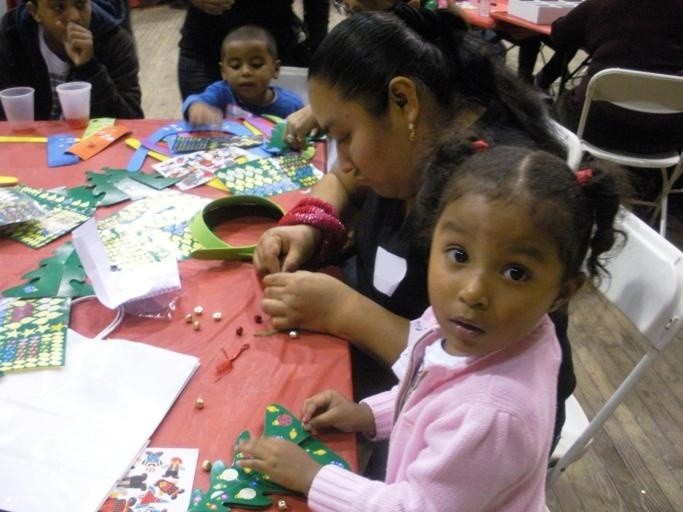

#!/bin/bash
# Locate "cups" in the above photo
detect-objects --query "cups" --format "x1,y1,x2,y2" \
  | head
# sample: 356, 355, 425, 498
0, 86, 36, 136
55, 81, 93, 131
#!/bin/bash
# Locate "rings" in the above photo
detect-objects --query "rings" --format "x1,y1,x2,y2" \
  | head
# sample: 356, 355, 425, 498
286, 133, 296, 146
295, 136, 304, 143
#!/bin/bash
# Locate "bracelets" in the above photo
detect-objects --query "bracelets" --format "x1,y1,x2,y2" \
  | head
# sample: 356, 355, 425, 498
278, 198, 348, 270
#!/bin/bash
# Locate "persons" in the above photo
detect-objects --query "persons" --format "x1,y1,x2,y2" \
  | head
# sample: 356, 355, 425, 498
228, 121, 635, 511
0, 1, 145, 122
279, 101, 318, 151
249, 1, 579, 479
283, 0, 331, 51
536, 0, 683, 222
164, 0, 309, 129
177, 23, 302, 130
340, 0, 387, 19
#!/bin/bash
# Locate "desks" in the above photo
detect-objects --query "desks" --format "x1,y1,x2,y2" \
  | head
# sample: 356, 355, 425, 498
0, 118, 357, 512
